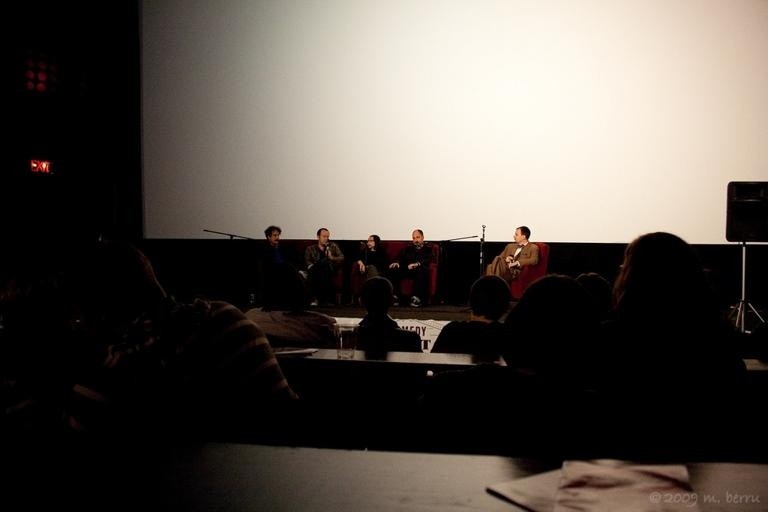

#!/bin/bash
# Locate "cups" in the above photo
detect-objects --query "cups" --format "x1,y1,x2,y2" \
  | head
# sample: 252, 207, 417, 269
335, 323, 358, 360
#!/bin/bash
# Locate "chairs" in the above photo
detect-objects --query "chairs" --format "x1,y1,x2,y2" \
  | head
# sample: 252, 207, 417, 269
484, 242, 549, 302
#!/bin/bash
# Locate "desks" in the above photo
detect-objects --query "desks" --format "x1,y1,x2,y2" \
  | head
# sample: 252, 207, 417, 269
1, 347, 766, 510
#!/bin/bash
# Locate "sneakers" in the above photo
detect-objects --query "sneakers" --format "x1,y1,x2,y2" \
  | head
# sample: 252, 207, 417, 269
410, 298, 422, 307
299, 270, 308, 280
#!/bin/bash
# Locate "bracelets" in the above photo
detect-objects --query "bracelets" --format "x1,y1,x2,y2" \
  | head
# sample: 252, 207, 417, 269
417, 262, 420, 267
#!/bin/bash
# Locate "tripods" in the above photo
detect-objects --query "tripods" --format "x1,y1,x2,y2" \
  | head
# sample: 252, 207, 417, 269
728, 242, 766, 335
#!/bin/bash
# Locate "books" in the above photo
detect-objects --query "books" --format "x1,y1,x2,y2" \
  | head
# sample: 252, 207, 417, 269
488, 459, 704, 512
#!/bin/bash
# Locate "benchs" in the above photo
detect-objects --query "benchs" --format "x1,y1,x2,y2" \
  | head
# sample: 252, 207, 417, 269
354, 240, 441, 305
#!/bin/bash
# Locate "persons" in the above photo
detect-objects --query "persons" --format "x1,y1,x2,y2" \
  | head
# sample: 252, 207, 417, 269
410, 273, 645, 461
241, 265, 342, 350
296, 228, 345, 306
604, 231, 767, 464
387, 230, 433, 307
338, 275, 426, 353
348, 234, 393, 282
0, 211, 300, 447
252, 225, 291, 277
490, 226, 540, 282
429, 274, 519, 354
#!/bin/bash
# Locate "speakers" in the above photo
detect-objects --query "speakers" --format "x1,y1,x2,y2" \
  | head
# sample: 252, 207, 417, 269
726, 182, 768, 244
